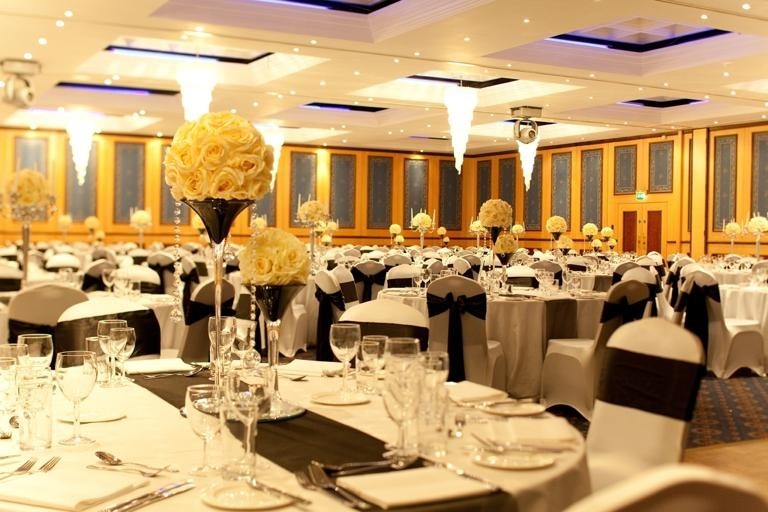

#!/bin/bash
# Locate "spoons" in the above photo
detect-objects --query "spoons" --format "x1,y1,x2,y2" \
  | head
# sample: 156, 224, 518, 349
95, 451, 180, 476
144, 365, 203, 377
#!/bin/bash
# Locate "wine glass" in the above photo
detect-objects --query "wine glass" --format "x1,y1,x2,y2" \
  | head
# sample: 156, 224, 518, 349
55, 263, 142, 307
376, 267, 604, 301
329, 324, 451, 464
699, 250, 752, 271
182, 313, 270, 478
1, 319, 142, 453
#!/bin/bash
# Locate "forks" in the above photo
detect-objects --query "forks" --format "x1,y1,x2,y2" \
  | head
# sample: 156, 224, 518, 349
296, 463, 371, 509
1, 456, 64, 473
0, 460, 35, 480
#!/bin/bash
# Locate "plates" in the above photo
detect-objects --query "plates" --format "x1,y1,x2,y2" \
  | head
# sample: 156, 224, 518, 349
478, 401, 544, 417
472, 450, 553, 469
311, 390, 372, 406
201, 479, 299, 511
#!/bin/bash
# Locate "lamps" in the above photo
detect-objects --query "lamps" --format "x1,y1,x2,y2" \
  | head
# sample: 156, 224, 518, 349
175, 42, 215, 120
0, 58, 42, 107
509, 105, 542, 145
444, 73, 480, 176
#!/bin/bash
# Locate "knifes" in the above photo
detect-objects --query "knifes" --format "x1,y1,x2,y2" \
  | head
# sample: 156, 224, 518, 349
109, 478, 196, 511
